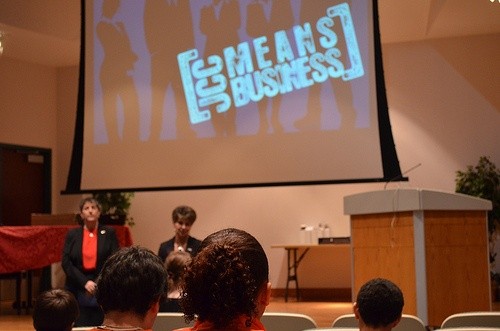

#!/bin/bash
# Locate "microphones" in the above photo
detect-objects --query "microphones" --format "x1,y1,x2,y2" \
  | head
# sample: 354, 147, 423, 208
384, 163, 421, 189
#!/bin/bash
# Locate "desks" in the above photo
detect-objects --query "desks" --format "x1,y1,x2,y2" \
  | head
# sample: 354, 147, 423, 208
0, 225, 133, 316
270, 243, 351, 302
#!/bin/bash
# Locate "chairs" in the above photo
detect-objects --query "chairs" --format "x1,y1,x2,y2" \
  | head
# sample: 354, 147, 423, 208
71, 297, 500, 331
26, 213, 79, 308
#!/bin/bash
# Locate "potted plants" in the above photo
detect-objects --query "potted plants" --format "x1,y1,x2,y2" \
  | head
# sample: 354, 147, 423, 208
93, 187, 136, 227
453, 156, 500, 302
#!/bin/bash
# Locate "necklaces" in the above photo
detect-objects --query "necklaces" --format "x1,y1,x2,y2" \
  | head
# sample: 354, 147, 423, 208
86, 225, 96, 237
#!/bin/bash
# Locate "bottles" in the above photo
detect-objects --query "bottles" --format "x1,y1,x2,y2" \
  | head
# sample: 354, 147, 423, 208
298, 222, 330, 245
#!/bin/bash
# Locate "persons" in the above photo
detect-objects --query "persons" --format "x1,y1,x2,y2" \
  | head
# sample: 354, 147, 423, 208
158, 206, 202, 312
353, 278, 405, 331
173, 228, 271, 331
61, 197, 119, 328
88, 246, 168, 331
32, 289, 78, 331
